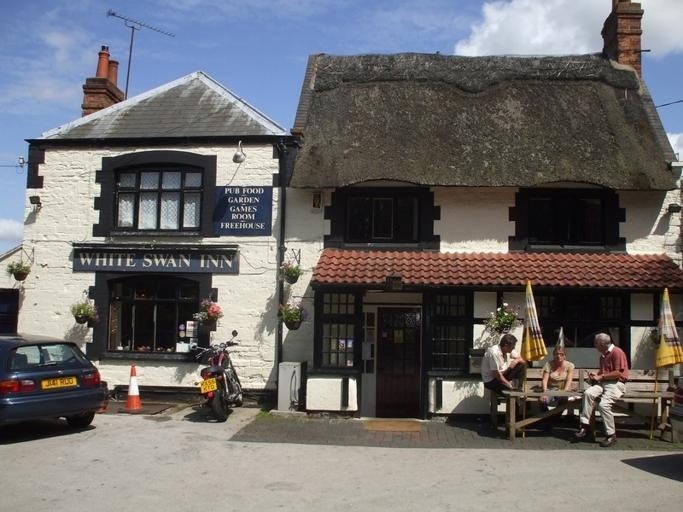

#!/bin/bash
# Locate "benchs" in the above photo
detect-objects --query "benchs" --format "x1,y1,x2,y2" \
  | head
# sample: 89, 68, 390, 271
489, 367, 675, 447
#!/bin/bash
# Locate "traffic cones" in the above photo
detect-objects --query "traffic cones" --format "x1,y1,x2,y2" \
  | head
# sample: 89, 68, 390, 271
119, 364, 148, 414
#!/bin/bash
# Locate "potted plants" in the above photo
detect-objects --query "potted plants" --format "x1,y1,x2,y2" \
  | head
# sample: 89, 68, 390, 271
7, 261, 31, 280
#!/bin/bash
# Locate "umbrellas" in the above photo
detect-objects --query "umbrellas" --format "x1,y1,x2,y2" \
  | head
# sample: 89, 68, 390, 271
649, 286, 683, 440
519, 277, 547, 436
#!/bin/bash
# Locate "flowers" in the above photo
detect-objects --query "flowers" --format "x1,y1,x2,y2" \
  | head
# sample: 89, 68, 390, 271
277, 303, 304, 322
282, 263, 300, 275
481, 302, 520, 329
192, 298, 224, 324
72, 302, 100, 321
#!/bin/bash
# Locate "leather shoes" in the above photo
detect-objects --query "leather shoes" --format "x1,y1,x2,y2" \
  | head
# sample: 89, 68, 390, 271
574, 426, 592, 438
599, 434, 616, 447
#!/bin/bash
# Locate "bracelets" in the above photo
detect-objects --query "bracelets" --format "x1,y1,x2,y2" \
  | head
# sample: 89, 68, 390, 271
601, 374, 605, 381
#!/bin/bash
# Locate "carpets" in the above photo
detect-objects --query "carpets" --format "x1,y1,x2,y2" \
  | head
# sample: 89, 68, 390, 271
365, 420, 422, 432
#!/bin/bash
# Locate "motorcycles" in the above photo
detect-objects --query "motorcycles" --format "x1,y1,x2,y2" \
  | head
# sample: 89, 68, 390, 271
191, 330, 244, 423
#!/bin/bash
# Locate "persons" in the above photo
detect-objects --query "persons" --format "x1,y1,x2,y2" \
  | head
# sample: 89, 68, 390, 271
481, 334, 524, 393
573, 332, 628, 447
537, 343, 575, 429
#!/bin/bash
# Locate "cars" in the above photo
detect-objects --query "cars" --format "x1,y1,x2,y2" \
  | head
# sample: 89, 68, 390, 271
1, 332, 109, 436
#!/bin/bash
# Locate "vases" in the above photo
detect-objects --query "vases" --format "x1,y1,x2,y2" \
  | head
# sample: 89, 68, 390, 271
75, 315, 89, 324
284, 274, 298, 283
283, 319, 302, 330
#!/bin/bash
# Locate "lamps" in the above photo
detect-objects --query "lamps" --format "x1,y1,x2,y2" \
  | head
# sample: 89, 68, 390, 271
233, 140, 248, 163
